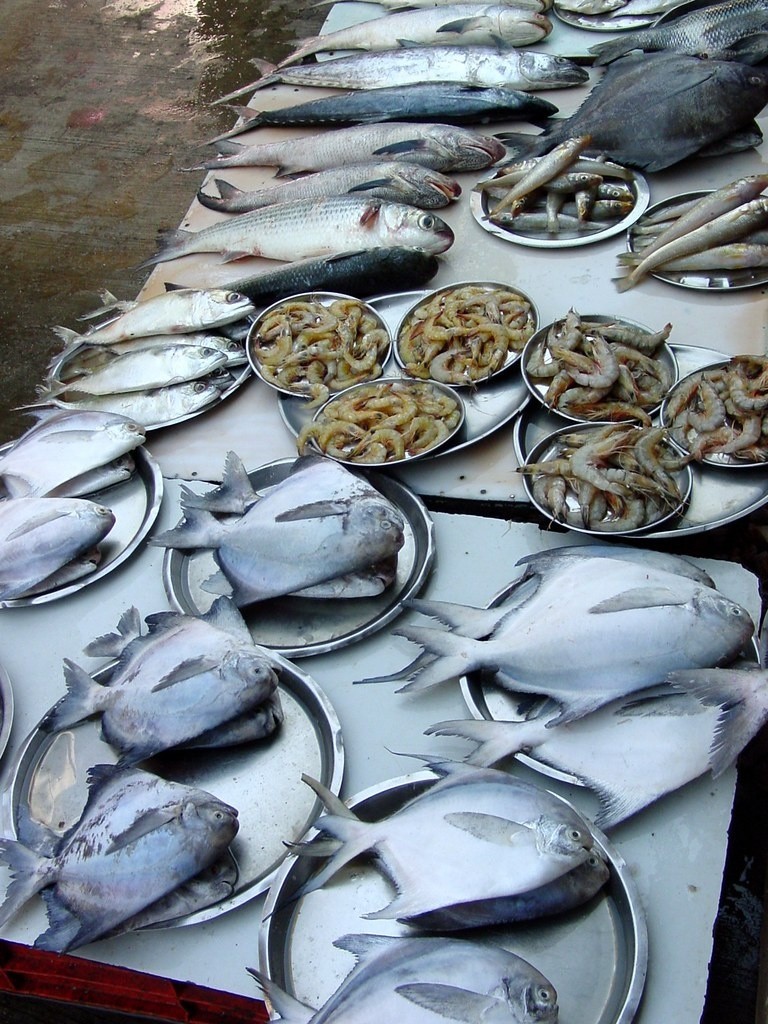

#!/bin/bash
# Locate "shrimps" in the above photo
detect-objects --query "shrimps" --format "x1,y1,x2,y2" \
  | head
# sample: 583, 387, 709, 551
248, 282, 768, 532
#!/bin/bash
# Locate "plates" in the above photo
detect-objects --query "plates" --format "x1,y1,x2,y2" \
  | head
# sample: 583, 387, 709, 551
459, 572, 764, 792
626, 187, 768, 290
46, 302, 258, 432
246, 286, 392, 394
0, 661, 14, 764
255, 769, 649, 1024
0, 431, 163, 607
391, 280, 541, 389
10, 639, 345, 931
660, 356, 767, 469
162, 455, 433, 659
521, 313, 678, 426
468, 157, 650, 247
306, 376, 465, 466
522, 420, 693, 536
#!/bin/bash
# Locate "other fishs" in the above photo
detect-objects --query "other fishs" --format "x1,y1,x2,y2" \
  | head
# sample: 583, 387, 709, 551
0, 0, 768, 1024
